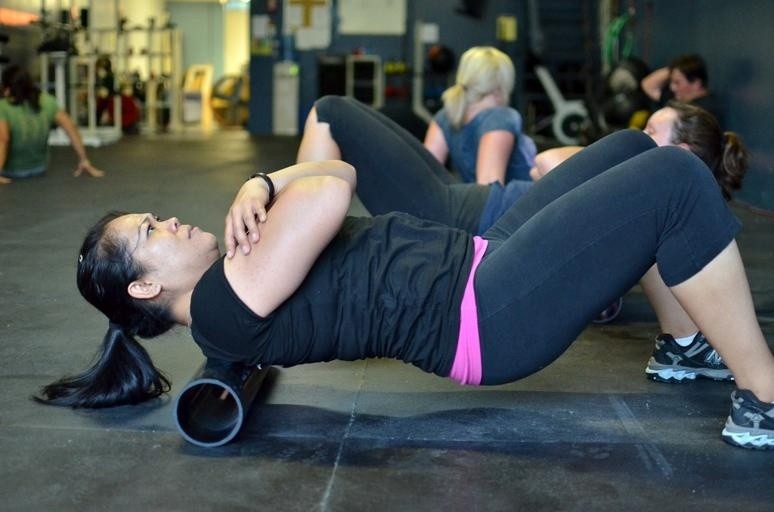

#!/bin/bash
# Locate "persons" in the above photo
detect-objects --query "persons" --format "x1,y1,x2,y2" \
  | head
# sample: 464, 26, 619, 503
34, 128, 774, 452
106, 84, 142, 135
0, 64, 106, 185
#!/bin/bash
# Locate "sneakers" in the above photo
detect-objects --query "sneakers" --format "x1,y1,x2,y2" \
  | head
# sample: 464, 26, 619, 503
645, 330, 736, 384
720, 388, 774, 449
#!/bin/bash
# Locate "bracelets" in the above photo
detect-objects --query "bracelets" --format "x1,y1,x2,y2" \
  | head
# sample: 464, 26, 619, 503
246, 172, 275, 209
